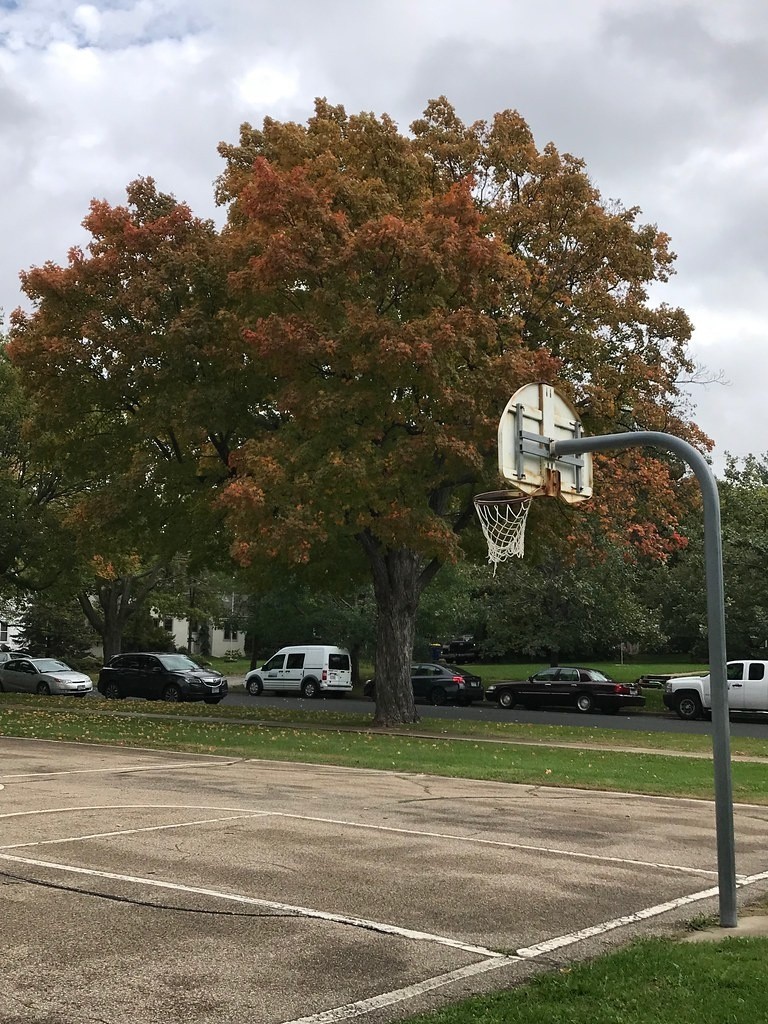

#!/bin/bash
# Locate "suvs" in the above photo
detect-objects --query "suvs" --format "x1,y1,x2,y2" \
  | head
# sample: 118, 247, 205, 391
98, 652, 231, 705
661, 659, 768, 720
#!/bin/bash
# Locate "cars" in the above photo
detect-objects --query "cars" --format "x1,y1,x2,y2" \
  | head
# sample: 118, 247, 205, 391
0, 658, 94, 697
0, 653, 34, 662
364, 662, 484, 705
484, 667, 647, 715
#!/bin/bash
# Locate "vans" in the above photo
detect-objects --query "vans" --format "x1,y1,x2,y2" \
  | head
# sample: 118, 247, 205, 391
243, 645, 354, 699
